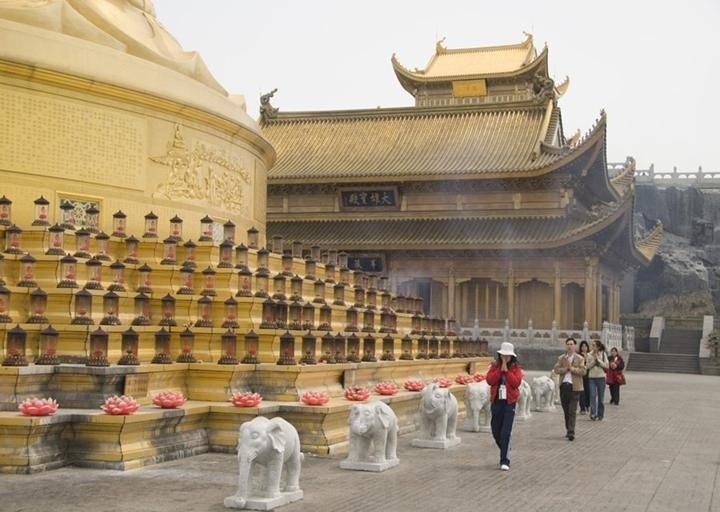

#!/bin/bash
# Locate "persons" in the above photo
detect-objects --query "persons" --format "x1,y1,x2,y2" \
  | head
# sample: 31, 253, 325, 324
553, 338, 588, 441
585, 340, 610, 422
577, 340, 591, 414
485, 342, 524, 470
606, 347, 625, 405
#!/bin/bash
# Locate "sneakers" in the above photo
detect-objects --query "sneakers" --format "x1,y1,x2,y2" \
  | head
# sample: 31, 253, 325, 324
610, 398, 621, 407
580, 407, 604, 422
501, 464, 510, 471
566, 435, 574, 441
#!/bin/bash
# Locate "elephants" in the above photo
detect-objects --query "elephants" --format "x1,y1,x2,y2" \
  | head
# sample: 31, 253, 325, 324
463, 379, 491, 432
514, 379, 532, 417
232, 414, 305, 510
345, 400, 398, 464
418, 380, 459, 442
550, 369, 563, 403
531, 376, 556, 411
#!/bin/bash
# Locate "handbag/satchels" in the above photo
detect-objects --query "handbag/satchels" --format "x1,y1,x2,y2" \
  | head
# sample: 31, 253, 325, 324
601, 367, 609, 374
616, 372, 627, 387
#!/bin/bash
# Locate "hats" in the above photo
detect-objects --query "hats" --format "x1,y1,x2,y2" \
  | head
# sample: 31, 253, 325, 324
495, 341, 517, 358
